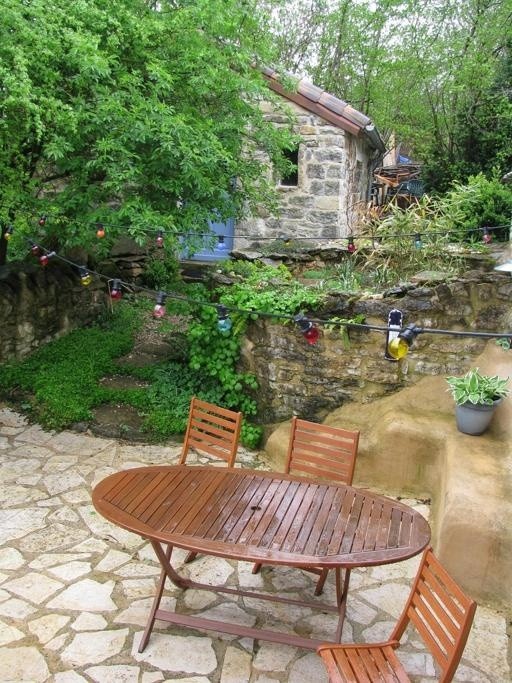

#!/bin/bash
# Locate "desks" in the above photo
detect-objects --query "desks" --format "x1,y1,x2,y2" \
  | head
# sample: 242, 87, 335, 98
93, 466, 431, 664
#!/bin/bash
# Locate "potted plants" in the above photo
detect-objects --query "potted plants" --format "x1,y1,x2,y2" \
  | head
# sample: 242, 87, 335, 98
443, 367, 512, 436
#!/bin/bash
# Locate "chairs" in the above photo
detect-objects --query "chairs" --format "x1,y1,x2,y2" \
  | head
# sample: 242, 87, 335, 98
316, 546, 477, 683
141, 398, 243, 563
252, 416, 360, 596
